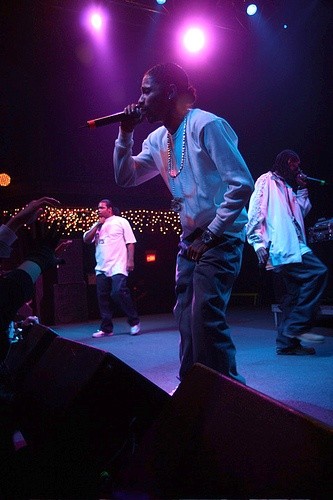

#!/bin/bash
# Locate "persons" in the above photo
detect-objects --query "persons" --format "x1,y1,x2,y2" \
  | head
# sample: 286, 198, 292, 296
83, 200, 140, 337
113, 61, 255, 396
246, 150, 328, 354
0, 197, 62, 321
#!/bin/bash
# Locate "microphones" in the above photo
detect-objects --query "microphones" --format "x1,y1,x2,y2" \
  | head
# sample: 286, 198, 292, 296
86, 106, 144, 130
299, 174, 325, 186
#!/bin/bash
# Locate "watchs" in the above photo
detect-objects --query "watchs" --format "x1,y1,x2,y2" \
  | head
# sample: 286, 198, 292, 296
202, 229, 219, 248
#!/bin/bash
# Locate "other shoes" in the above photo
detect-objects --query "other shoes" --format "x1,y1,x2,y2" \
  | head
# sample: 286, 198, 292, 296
282, 331, 325, 343
276, 343, 316, 355
130, 322, 141, 334
92, 329, 113, 338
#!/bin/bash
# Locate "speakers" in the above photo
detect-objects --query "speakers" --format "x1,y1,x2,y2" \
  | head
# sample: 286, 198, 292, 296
0, 321, 59, 390
49, 238, 84, 285
307, 239, 333, 303
45, 282, 88, 324
0, 336, 171, 500
106, 363, 333, 500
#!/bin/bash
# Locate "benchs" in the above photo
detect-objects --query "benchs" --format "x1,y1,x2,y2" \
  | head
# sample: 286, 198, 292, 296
271, 304, 333, 328
230, 293, 262, 305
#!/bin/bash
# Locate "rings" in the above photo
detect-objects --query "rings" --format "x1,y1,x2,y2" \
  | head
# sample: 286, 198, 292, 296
192, 257, 195, 260
